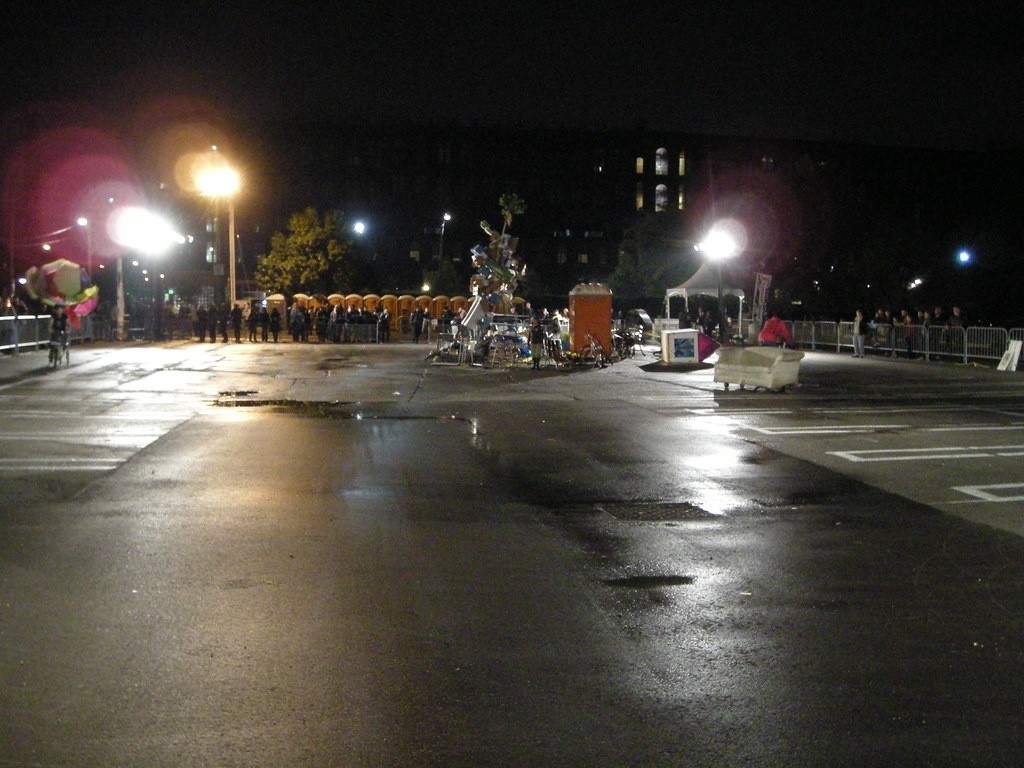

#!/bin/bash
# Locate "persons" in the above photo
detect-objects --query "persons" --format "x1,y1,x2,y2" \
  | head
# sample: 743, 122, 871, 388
442, 306, 467, 333
197, 303, 281, 342
679, 305, 715, 336
409, 306, 431, 343
165, 302, 193, 340
289, 302, 392, 344
0, 295, 28, 354
528, 318, 543, 370
48, 306, 70, 362
873, 306, 965, 361
851, 309, 868, 358
759, 311, 795, 347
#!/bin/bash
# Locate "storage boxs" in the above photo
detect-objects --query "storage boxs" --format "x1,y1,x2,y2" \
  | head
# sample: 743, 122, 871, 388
661, 329, 700, 364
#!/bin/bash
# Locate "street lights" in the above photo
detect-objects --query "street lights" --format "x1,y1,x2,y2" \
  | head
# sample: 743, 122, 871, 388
192, 162, 236, 313
77, 216, 92, 277
705, 231, 732, 343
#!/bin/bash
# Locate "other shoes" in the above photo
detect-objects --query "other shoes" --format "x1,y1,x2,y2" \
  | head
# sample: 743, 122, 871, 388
852, 354, 859, 358
861, 354, 864, 358
49, 362, 55, 368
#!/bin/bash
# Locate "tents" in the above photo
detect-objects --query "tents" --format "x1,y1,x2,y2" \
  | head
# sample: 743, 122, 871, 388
666, 264, 745, 333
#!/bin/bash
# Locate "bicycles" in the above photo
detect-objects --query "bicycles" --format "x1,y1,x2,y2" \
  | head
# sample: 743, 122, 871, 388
578, 331, 606, 369
49, 330, 69, 369
619, 329, 631, 359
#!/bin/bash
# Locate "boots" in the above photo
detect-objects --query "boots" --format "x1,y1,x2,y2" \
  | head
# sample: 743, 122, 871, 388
536, 358, 542, 371
531, 358, 537, 370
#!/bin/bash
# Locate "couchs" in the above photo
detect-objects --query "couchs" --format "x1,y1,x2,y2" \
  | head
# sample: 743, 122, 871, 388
713, 346, 805, 394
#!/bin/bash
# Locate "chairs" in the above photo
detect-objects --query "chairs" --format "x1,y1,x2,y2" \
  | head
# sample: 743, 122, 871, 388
543, 338, 573, 369
